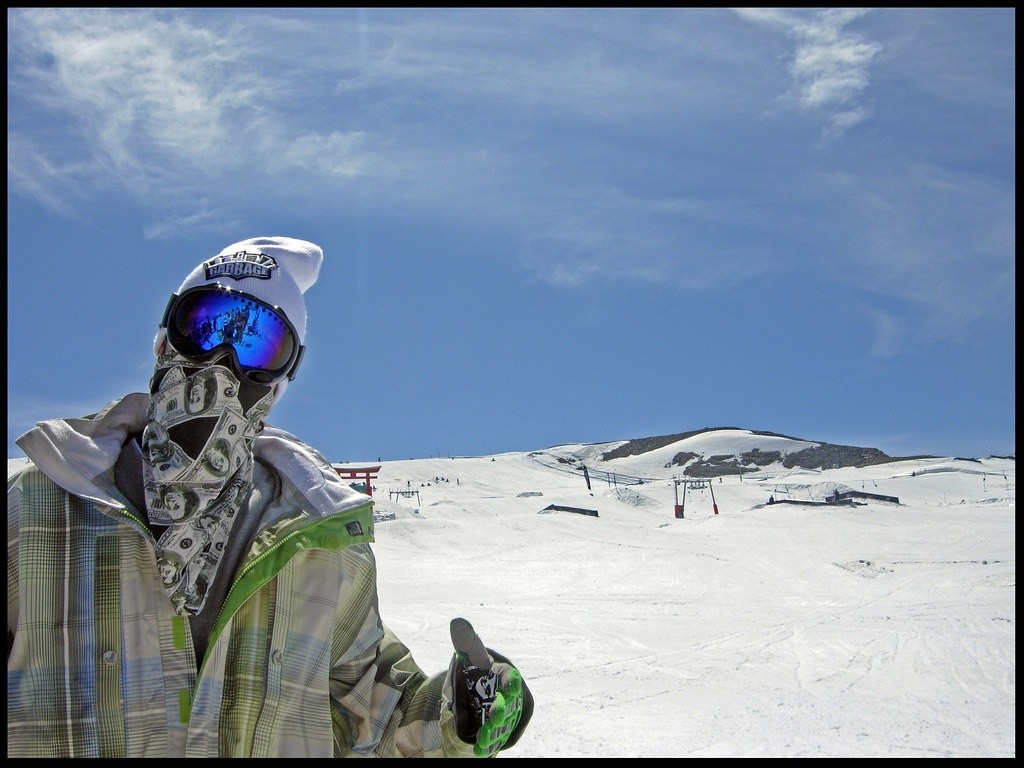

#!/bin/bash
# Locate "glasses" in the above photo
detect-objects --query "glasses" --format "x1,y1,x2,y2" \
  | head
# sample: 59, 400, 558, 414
160, 283, 306, 388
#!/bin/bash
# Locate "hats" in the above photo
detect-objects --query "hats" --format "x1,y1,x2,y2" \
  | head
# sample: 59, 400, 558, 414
153, 236, 324, 405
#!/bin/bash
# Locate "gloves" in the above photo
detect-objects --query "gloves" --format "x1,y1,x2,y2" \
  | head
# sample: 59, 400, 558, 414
450, 618, 524, 758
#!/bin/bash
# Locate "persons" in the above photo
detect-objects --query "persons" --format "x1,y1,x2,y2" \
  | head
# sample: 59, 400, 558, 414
8, 236, 534, 758
200, 305, 249, 345
